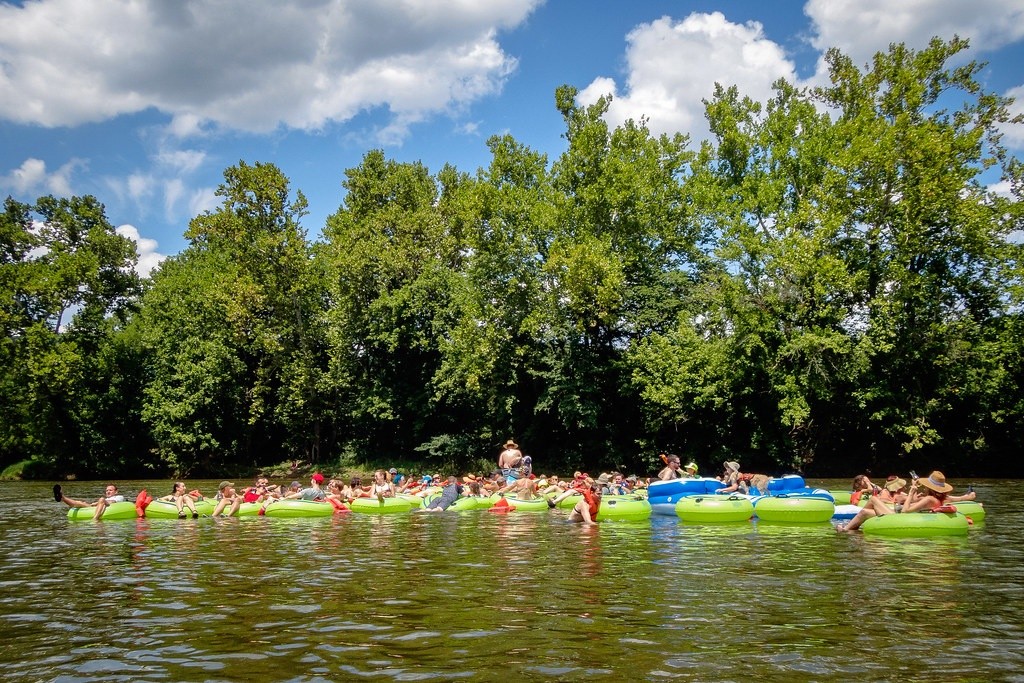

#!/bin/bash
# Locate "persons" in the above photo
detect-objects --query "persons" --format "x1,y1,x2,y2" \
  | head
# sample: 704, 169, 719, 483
371, 467, 445, 500
662, 454, 681, 481
463, 469, 516, 497
287, 474, 326, 500
837, 471, 976, 531
569, 484, 602, 524
499, 440, 522, 476
159, 482, 199, 518
426, 476, 469, 512
201, 478, 303, 517
715, 461, 747, 495
503, 456, 657, 507
326, 480, 350, 503
53, 483, 123, 519
676, 462, 702, 479
346, 477, 372, 497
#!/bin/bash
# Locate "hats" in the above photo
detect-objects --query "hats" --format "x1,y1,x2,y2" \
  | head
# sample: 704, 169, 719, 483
503, 440, 518, 449
491, 469, 501, 474
626, 476, 637, 486
595, 473, 609, 484
219, 481, 234, 489
389, 468, 397, 474
463, 475, 478, 482
433, 474, 439, 479
723, 461, 740, 472
310, 474, 324, 485
919, 471, 955, 490
291, 481, 302, 488
884, 476, 906, 491
685, 462, 698, 471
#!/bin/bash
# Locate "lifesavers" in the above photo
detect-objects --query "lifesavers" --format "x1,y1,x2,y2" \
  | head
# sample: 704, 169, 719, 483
754, 496, 836, 523
65, 489, 655, 522
829, 490, 985, 535
674, 494, 754, 523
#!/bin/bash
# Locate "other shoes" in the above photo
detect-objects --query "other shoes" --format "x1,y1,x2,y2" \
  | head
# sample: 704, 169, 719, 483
203, 513, 209, 518
178, 511, 186, 518
192, 511, 198, 518
220, 514, 227, 518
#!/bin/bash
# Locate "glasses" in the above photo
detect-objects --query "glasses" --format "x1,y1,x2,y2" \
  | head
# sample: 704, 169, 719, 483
673, 462, 679, 465
391, 473, 396, 475
